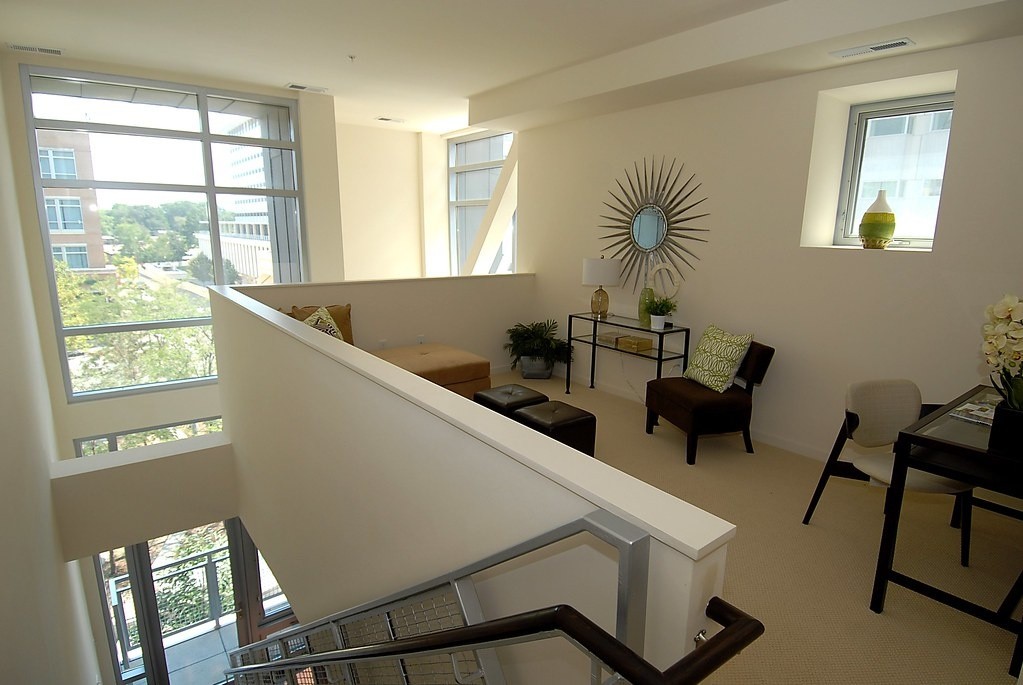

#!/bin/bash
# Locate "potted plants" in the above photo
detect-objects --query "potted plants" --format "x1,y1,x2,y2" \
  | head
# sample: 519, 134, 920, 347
503, 319, 573, 379
646, 296, 678, 329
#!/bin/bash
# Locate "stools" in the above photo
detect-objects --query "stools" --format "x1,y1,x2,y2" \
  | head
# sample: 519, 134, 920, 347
473, 384, 549, 419
513, 401, 597, 459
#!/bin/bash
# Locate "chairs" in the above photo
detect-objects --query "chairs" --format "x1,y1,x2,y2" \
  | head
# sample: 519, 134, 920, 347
645, 342, 775, 465
803, 379, 976, 568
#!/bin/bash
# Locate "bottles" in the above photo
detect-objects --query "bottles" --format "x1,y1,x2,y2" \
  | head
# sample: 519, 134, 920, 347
858, 190, 895, 249
637, 288, 654, 327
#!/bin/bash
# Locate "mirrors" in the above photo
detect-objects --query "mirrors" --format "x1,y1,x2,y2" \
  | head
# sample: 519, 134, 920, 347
598, 154, 709, 293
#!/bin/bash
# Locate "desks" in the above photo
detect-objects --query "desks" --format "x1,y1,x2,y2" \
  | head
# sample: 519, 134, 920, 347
566, 312, 690, 394
870, 385, 1023, 679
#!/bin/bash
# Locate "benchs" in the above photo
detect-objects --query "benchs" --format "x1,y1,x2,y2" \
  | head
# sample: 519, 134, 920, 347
369, 343, 491, 400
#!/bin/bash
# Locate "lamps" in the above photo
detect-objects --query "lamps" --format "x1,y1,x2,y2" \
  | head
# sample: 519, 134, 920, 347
582, 255, 621, 318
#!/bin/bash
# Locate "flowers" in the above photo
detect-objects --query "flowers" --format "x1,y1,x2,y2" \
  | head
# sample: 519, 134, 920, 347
981, 295, 1023, 410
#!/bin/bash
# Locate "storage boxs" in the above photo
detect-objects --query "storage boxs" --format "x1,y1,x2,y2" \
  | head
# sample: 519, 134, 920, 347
597, 331, 653, 350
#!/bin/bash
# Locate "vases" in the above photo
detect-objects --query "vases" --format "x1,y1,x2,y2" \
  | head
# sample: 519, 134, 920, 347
859, 189, 895, 250
986, 400, 1023, 459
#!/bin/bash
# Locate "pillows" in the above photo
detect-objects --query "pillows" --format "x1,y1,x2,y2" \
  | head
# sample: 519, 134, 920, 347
281, 303, 352, 344
684, 325, 753, 394
